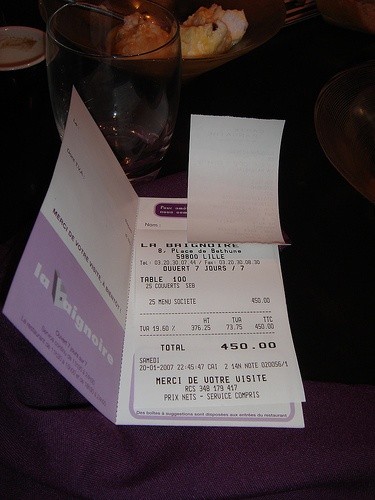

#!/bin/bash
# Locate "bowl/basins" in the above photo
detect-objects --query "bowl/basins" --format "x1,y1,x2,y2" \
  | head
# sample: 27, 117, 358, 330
315, 60, 375, 206
52, 0, 286, 79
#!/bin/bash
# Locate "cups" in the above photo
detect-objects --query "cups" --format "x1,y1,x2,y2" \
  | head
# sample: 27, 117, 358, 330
45, 0, 182, 188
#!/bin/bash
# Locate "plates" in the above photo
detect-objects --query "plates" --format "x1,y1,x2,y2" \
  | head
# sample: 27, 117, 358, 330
0, 25, 50, 71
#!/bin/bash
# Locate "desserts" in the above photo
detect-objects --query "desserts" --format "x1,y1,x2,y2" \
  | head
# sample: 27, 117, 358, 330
109, 4, 249, 61
